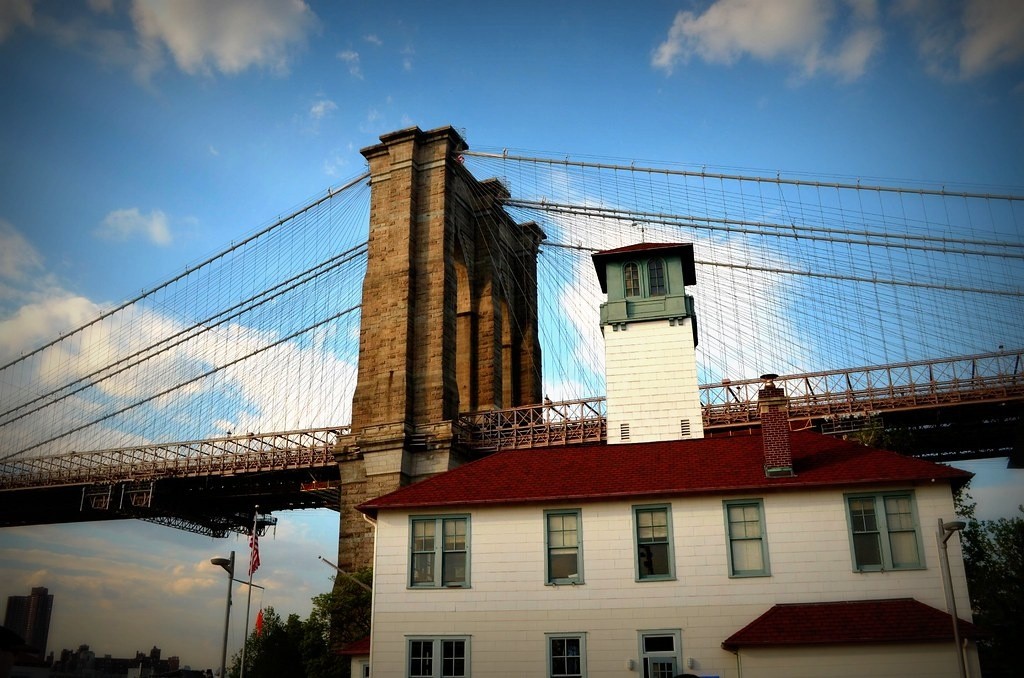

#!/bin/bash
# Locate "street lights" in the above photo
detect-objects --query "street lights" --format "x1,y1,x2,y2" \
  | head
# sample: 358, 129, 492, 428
210, 551, 236, 677
935, 517, 968, 678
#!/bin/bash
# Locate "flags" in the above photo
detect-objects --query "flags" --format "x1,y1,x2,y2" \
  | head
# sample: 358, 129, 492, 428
256, 609, 263, 637
248, 536, 260, 575
